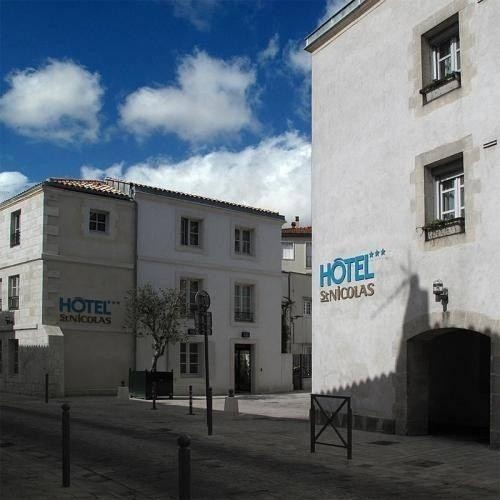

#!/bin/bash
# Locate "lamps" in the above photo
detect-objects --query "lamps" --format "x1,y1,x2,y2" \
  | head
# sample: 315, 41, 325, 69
5, 315, 13, 325
432, 279, 448, 312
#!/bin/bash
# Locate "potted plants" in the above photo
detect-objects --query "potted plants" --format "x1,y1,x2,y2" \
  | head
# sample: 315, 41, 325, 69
120, 279, 196, 403
